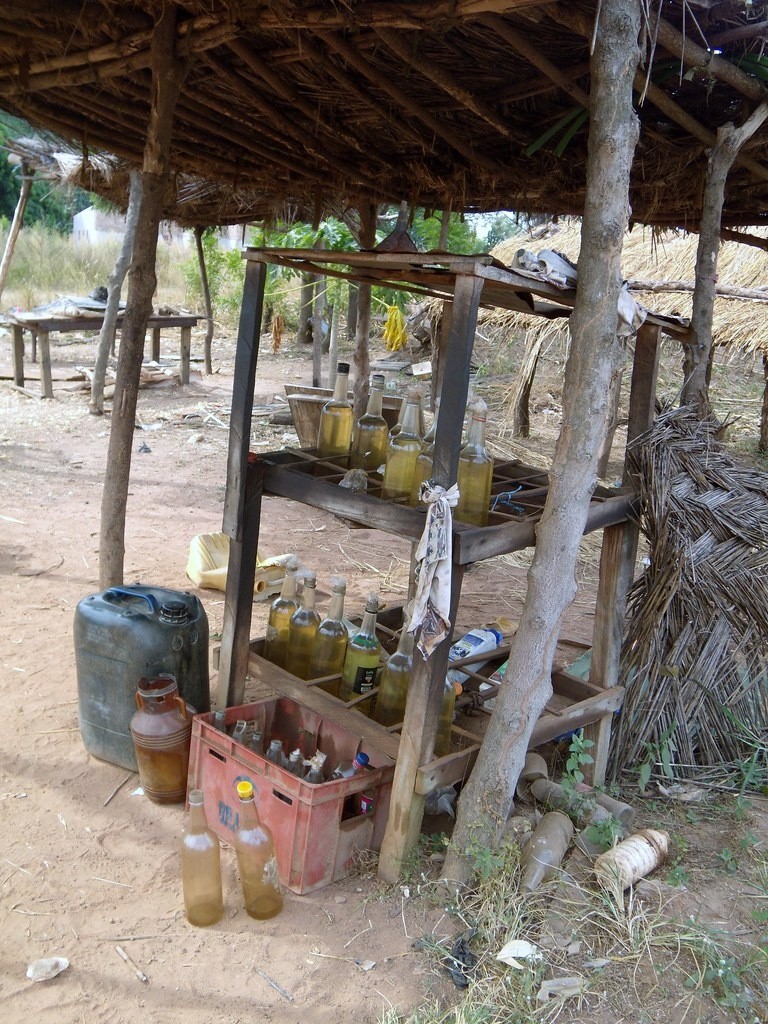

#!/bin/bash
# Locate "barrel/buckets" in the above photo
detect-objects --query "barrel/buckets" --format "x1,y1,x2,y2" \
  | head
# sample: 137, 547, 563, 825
74, 583, 209, 773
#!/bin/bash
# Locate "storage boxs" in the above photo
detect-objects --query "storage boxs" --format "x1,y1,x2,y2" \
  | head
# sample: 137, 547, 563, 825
189, 692, 394, 894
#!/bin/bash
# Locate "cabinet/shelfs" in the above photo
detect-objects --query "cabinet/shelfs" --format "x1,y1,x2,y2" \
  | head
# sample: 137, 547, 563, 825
210, 246, 686, 887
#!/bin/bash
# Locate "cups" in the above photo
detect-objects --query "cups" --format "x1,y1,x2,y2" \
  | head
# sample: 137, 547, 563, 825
516, 752, 549, 805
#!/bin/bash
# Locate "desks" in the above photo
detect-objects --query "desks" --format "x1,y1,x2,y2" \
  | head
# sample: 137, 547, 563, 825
5, 303, 206, 398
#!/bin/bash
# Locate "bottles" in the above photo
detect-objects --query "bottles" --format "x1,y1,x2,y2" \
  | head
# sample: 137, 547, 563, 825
531, 779, 610, 827
210, 709, 377, 815
574, 782, 635, 828
316, 361, 494, 528
262, 562, 503, 759
593, 828, 671, 892
234, 781, 284, 920
179, 789, 224, 927
518, 813, 574, 896
